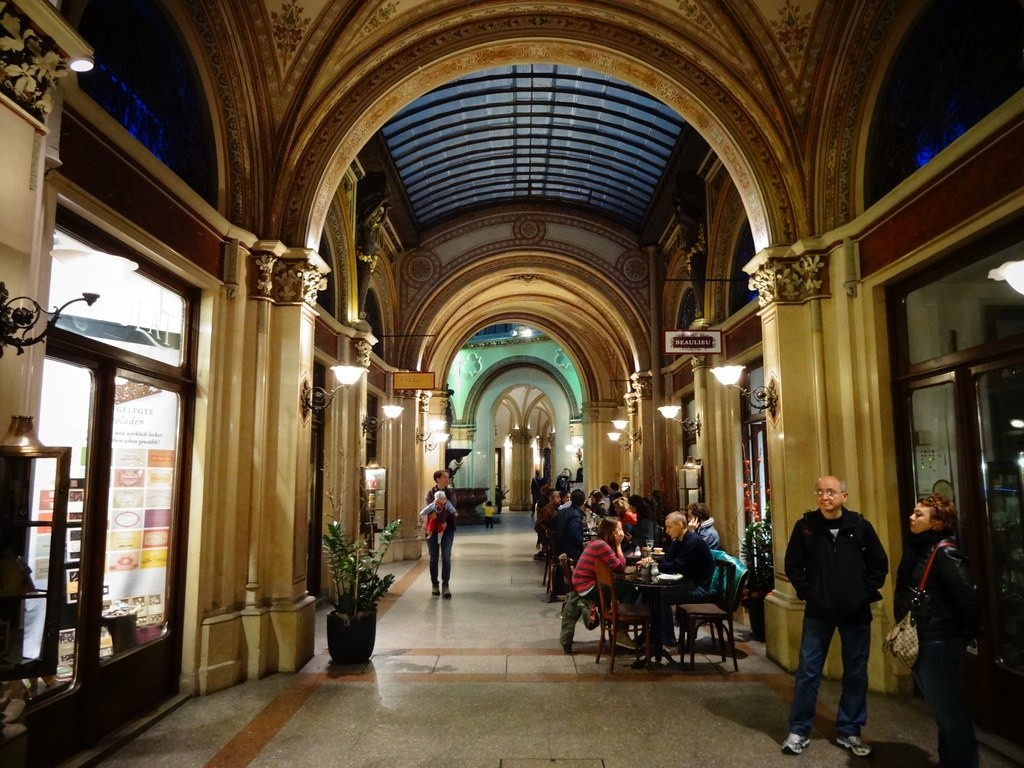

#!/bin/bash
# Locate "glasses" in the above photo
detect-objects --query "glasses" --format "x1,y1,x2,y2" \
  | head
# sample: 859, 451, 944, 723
813, 490, 844, 496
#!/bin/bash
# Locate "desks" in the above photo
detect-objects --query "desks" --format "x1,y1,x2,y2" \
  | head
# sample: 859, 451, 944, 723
614, 576, 681, 670
623, 544, 665, 566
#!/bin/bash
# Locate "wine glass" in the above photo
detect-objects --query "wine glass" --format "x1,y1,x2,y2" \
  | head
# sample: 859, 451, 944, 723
586, 518, 594, 535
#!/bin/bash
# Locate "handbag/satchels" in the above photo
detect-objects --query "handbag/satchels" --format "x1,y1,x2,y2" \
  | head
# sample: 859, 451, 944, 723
883, 612, 919, 669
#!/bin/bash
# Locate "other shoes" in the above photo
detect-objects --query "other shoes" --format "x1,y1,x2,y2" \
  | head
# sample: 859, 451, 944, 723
442, 582, 451, 598
432, 580, 441, 595
662, 646, 680, 655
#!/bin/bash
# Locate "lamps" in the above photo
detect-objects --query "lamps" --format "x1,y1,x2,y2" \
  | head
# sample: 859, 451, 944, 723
416, 420, 450, 452
657, 406, 701, 436
0, 249, 139, 357
607, 432, 631, 451
611, 420, 641, 444
362, 405, 404, 436
709, 365, 778, 417
301, 365, 364, 418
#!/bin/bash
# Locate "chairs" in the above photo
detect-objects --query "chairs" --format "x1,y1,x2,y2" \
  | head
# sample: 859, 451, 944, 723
535, 519, 738, 674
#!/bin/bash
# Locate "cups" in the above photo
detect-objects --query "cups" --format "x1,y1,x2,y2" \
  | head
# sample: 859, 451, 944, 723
654, 548, 663, 553
646, 540, 654, 548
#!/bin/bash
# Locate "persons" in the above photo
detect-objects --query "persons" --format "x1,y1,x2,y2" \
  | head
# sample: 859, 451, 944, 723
483, 501, 495, 528
534, 485, 720, 656
531, 470, 543, 511
419, 470, 459, 597
576, 468, 583, 481
782, 476, 889, 757
893, 492, 985, 768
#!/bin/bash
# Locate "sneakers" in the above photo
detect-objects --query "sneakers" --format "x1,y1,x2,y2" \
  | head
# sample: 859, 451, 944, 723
615, 631, 636, 650
781, 732, 810, 755
836, 736, 871, 756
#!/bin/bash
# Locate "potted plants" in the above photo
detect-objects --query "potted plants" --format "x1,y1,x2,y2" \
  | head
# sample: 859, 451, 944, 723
495, 486, 509, 513
737, 519, 775, 641
319, 517, 402, 665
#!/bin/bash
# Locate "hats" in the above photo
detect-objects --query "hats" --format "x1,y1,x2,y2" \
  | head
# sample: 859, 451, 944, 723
435, 490, 447, 501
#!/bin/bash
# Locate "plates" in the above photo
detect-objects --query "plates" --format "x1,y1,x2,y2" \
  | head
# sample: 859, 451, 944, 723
652, 552, 665, 555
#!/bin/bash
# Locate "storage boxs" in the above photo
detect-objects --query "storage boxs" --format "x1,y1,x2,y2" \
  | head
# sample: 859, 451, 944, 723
679, 489, 701, 510
678, 464, 701, 489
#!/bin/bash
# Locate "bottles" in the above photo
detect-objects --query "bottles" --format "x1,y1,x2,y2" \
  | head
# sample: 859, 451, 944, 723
641, 547, 652, 557
634, 545, 641, 557
651, 563, 659, 575
607, 495, 610, 503
